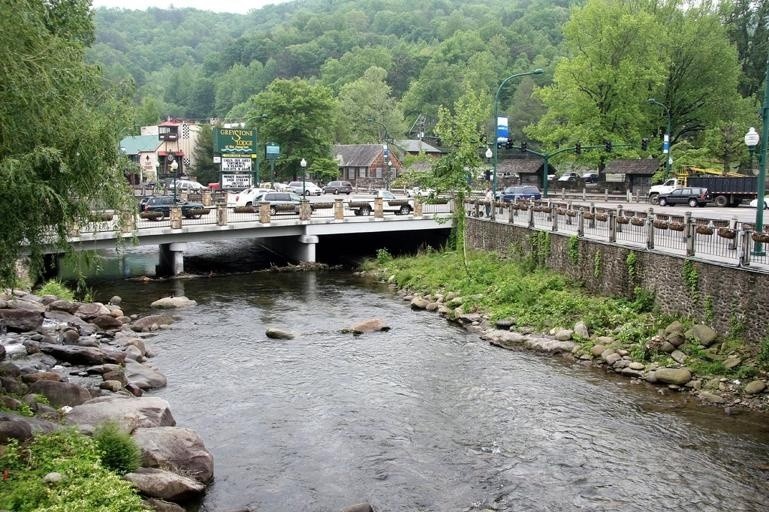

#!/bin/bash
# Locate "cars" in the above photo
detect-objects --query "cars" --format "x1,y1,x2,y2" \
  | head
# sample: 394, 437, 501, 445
140, 179, 352, 222
406, 187, 436, 197
495, 185, 540, 200
548, 171, 596, 186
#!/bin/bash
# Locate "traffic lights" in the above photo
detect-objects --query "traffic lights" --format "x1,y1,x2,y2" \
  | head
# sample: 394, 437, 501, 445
497, 144, 502, 150
575, 143, 580, 153
521, 141, 527, 152
508, 137, 512, 149
642, 139, 647, 150
605, 142, 611, 152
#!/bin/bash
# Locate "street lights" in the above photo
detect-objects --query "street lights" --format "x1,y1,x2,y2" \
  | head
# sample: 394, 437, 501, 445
485, 148, 492, 167
171, 160, 179, 199
493, 69, 544, 198
300, 159, 306, 198
369, 119, 389, 190
649, 98, 672, 176
388, 160, 393, 189
744, 127, 760, 175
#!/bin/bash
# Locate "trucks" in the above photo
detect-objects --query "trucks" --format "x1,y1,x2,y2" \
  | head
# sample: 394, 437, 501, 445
647, 175, 769, 207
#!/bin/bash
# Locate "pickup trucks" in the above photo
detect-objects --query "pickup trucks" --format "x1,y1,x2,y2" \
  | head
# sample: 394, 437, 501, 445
346, 190, 414, 215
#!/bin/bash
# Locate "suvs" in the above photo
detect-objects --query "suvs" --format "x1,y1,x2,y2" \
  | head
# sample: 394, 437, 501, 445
658, 187, 712, 207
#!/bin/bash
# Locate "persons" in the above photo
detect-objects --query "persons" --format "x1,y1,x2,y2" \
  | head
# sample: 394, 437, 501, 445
484, 188, 492, 218
